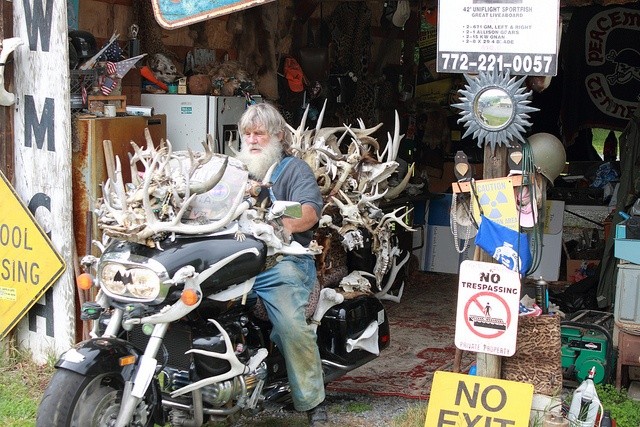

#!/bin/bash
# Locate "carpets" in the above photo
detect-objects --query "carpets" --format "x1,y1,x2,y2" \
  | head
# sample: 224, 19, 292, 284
318, 269, 472, 399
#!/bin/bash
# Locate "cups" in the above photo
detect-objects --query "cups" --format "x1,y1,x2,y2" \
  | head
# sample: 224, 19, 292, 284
103, 105, 117, 118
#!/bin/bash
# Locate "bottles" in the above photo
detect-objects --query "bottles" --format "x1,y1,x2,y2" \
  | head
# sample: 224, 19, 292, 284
536, 275, 550, 314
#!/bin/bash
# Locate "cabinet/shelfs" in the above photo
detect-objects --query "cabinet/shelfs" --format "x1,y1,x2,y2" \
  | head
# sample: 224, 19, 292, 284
73, 113, 169, 343
610, 219, 640, 392
425, 192, 459, 278
562, 202, 608, 243
526, 199, 565, 284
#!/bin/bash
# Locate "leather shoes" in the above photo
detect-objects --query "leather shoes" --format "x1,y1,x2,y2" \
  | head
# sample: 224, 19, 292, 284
310, 404, 331, 426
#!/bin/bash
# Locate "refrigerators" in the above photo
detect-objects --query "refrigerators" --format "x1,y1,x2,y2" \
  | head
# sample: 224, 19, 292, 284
140, 93, 262, 156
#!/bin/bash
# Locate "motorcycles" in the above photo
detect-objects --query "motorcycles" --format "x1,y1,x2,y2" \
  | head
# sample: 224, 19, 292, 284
34, 229, 389, 425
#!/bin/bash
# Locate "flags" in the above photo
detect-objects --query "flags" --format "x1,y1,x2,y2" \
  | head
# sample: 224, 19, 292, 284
104, 60, 118, 76
99, 40, 124, 61
101, 77, 117, 96
114, 54, 143, 79
80, 83, 87, 108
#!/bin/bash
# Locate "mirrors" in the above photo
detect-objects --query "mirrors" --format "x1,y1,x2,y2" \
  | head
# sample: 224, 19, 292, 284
449, 60, 541, 155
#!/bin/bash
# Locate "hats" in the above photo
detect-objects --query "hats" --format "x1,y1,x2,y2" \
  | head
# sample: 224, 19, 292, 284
507, 170, 538, 227
450, 177, 479, 239
393, 1, 411, 27
284, 58, 304, 92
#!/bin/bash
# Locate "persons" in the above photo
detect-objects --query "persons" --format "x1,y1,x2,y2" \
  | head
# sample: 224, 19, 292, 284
235, 101, 330, 427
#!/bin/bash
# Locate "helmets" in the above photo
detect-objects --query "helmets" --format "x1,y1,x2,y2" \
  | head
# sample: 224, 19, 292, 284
527, 133, 566, 185
